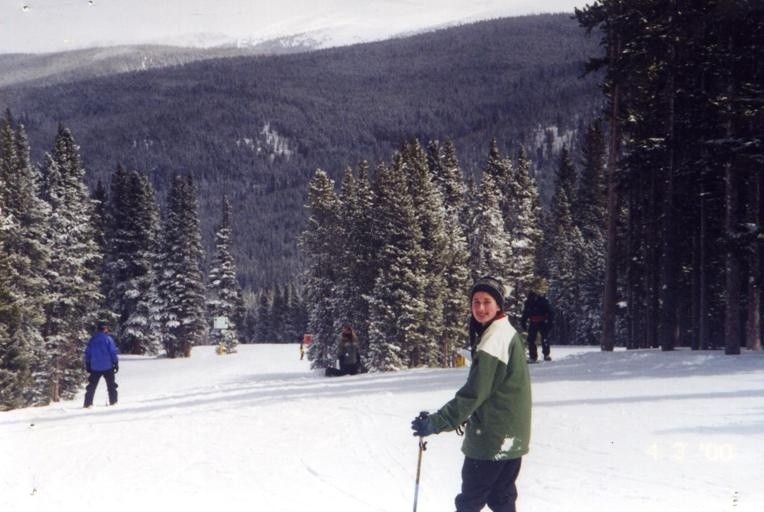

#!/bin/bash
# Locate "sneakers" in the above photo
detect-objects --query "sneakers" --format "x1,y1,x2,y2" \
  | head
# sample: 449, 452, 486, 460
528, 355, 551, 363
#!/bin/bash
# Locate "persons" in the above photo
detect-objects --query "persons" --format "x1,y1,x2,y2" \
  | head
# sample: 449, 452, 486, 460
300, 341, 304, 361
411, 276, 532, 512
84, 321, 119, 406
520, 291, 555, 363
333, 323, 361, 374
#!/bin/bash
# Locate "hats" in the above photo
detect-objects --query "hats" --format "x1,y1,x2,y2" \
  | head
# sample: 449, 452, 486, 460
471, 276, 505, 311
96, 320, 110, 326
342, 328, 352, 338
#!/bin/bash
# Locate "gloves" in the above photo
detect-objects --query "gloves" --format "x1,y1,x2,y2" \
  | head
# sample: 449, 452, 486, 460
87, 362, 90, 373
412, 415, 441, 435
113, 361, 119, 373
